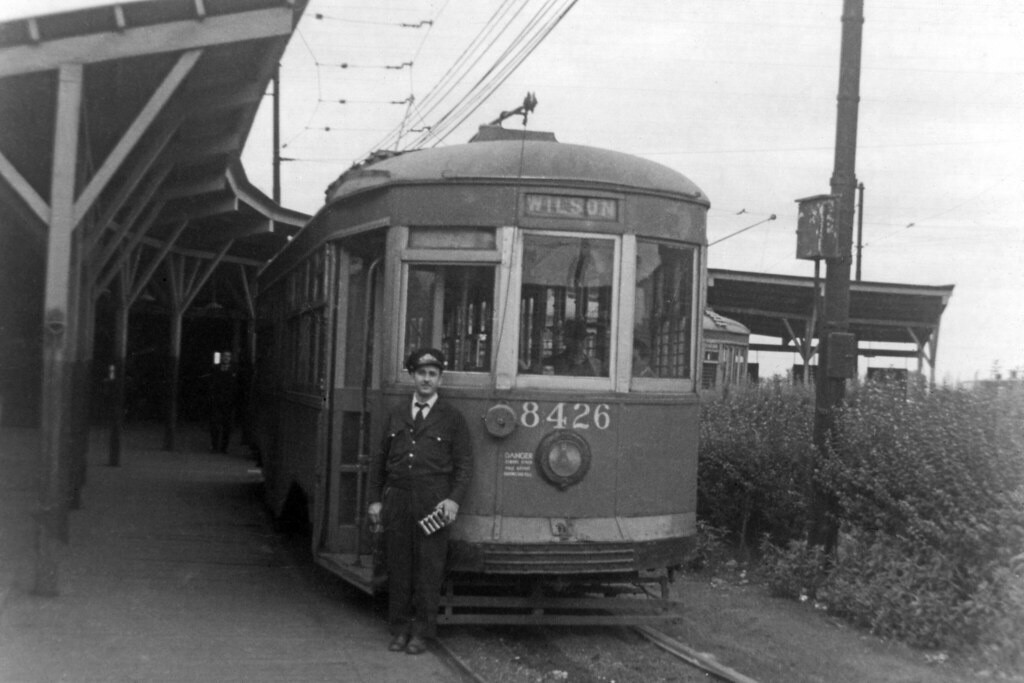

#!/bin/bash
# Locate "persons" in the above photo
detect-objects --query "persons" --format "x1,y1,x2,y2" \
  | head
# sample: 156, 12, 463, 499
542, 320, 607, 376
629, 337, 657, 377
368, 346, 473, 655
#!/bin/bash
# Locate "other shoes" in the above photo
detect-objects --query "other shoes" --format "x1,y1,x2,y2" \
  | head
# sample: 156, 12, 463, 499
389, 632, 410, 652
404, 638, 428, 654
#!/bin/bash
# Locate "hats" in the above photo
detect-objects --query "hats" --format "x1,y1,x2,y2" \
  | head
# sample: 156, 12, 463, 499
406, 347, 446, 376
634, 323, 652, 348
559, 319, 594, 338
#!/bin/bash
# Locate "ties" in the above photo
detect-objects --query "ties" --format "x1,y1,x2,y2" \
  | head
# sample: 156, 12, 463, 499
413, 402, 429, 432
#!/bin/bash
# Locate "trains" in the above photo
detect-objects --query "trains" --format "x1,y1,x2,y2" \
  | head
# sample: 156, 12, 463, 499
254, 92, 708, 626
